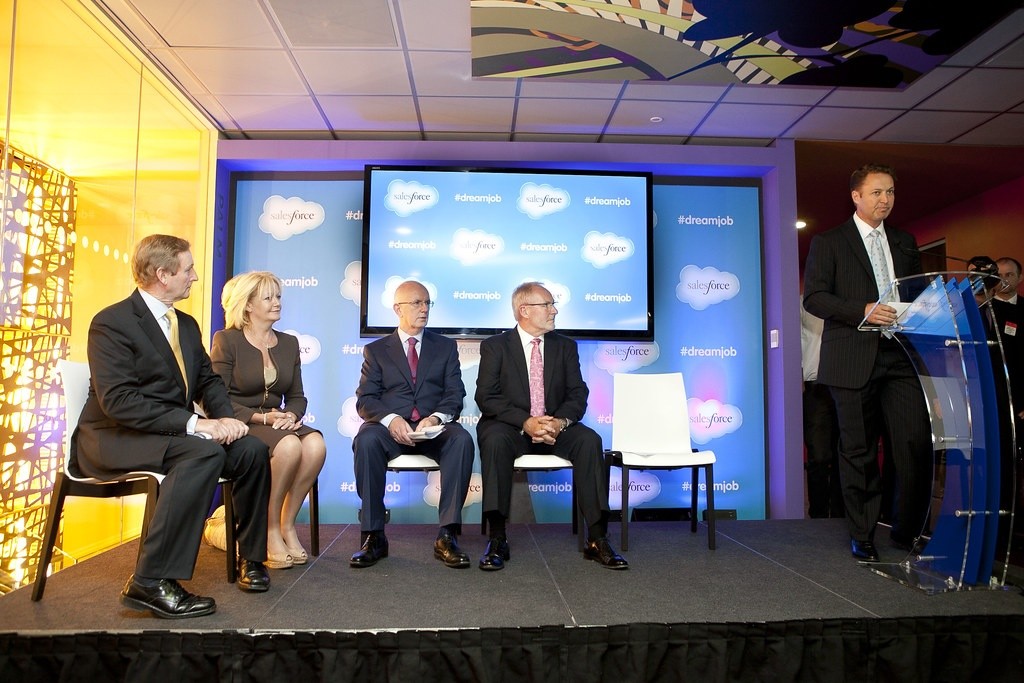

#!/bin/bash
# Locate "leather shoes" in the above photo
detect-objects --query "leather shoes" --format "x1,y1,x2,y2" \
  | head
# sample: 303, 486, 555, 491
848, 537, 879, 562
891, 533, 933, 555
118, 573, 217, 618
352, 535, 389, 568
433, 536, 470, 566
235, 555, 271, 591
479, 538, 510, 571
581, 537, 628, 569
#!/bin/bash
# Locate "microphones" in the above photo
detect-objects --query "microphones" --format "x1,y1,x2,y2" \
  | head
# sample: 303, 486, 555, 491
906, 247, 996, 270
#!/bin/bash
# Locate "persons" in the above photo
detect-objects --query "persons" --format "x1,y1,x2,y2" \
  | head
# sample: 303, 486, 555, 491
69, 235, 272, 619
349, 280, 475, 569
799, 294, 893, 519
199, 271, 327, 568
802, 162, 932, 564
995, 257, 1024, 307
967, 256, 999, 304
474, 282, 630, 570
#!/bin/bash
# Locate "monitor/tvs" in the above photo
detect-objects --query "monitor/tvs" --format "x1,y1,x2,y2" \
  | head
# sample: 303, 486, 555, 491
359, 165, 655, 343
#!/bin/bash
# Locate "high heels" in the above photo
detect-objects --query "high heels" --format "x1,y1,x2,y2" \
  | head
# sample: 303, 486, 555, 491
288, 544, 307, 565
262, 547, 292, 568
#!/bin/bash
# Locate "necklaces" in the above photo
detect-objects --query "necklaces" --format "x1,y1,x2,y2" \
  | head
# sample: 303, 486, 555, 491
249, 329, 272, 351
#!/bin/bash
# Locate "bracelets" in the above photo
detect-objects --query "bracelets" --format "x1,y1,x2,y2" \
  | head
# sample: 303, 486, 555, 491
264, 413, 266, 425
559, 418, 566, 432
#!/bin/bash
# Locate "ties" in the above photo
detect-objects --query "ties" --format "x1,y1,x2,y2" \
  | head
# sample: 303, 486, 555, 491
870, 229, 896, 339
165, 310, 189, 399
529, 339, 546, 418
406, 338, 419, 420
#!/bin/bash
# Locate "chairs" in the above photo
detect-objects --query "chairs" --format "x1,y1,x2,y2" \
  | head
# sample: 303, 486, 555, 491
604, 372, 717, 551
30, 359, 237, 602
482, 454, 579, 536
387, 453, 440, 474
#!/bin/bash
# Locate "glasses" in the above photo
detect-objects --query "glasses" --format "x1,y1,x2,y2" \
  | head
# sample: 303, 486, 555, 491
523, 302, 559, 310
400, 301, 433, 309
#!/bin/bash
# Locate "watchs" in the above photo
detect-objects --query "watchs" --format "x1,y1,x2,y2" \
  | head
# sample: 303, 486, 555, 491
432, 416, 442, 425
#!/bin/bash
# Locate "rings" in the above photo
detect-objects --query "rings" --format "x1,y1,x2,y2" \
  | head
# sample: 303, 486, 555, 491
290, 420, 292, 423
